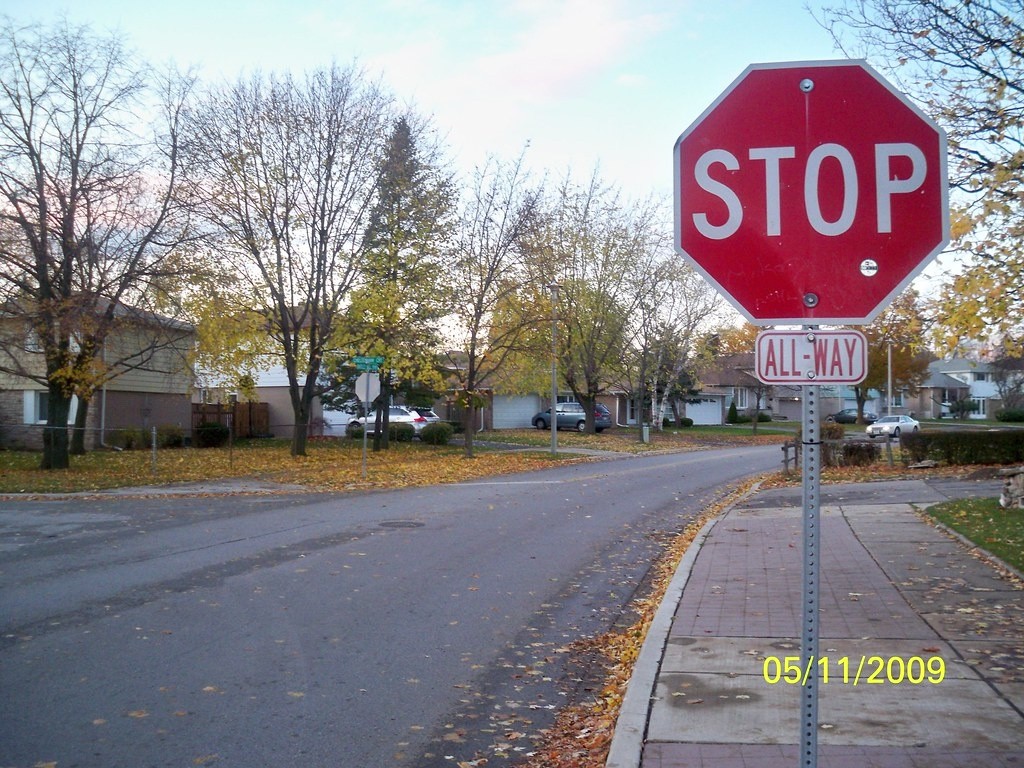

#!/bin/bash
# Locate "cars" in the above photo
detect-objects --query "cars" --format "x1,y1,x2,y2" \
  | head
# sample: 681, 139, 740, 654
866, 415, 921, 438
825, 408, 878, 424
345, 405, 440, 437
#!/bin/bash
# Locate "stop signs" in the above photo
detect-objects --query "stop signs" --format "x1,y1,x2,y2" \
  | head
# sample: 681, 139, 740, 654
674, 58, 950, 326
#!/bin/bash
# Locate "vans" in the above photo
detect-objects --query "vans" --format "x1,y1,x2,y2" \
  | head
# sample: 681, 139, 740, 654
532, 402, 612, 433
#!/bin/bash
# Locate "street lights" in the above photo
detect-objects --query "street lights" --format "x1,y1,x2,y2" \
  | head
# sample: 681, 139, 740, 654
886, 340, 895, 416
